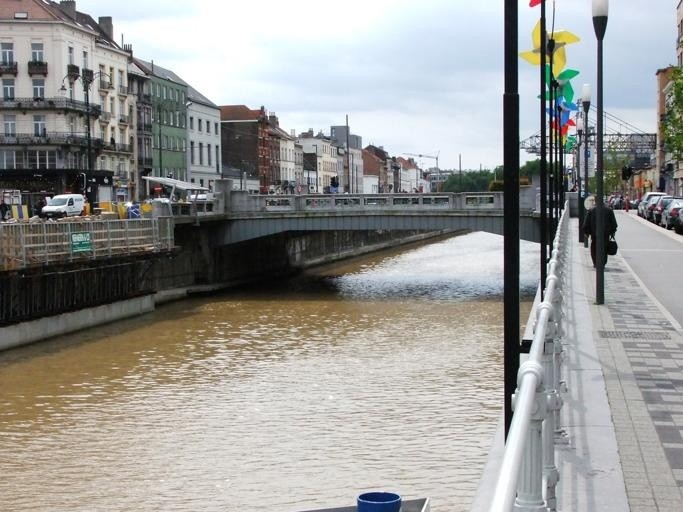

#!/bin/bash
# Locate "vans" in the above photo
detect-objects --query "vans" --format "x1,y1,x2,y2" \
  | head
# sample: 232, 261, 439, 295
41, 193, 84, 218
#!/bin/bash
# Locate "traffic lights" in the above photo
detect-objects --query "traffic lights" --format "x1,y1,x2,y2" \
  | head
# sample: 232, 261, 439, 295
622, 165, 633, 180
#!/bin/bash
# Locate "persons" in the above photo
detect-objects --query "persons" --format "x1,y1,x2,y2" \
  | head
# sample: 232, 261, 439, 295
582, 196, 618, 269
0, 200, 7, 221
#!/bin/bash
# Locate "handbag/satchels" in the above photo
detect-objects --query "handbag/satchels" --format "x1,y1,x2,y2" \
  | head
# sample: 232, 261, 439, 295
608, 235, 617, 256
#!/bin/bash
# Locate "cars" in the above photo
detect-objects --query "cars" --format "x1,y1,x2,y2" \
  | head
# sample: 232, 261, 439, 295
637, 192, 683, 234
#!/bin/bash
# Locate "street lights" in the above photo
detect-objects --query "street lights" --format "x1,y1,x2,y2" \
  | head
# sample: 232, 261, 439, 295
60, 71, 115, 215
581, 84, 591, 198
591, 0, 609, 305
576, 118, 583, 198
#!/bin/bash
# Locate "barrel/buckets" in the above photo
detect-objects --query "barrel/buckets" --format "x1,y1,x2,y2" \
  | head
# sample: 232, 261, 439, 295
357, 491, 403, 512
